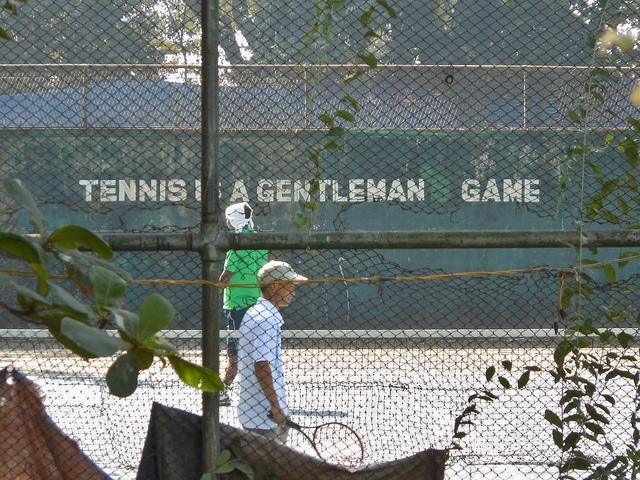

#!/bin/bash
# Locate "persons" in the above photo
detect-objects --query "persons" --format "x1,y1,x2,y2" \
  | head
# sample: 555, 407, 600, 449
236, 260, 309, 445
217, 200, 269, 407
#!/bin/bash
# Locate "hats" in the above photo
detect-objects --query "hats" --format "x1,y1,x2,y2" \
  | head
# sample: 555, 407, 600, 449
258, 260, 309, 287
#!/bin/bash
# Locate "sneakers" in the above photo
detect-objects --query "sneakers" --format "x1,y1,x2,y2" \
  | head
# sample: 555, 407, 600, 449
219, 390, 231, 406
